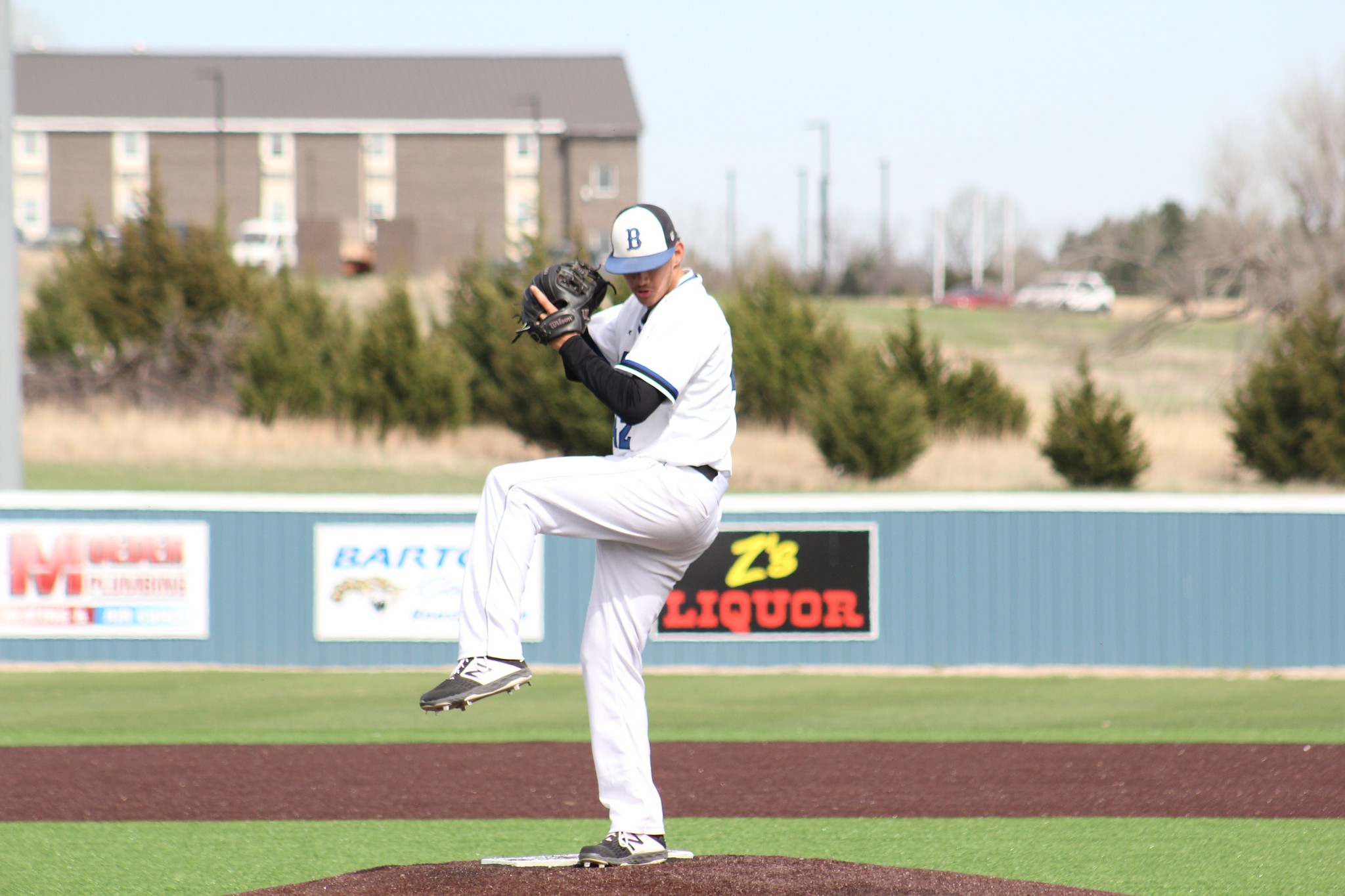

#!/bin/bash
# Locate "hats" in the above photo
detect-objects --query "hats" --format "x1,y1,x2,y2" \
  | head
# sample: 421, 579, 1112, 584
339, 237, 374, 272
604, 203, 678, 276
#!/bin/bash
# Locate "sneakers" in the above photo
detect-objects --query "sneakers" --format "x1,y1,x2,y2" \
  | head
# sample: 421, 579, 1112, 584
578, 832, 668, 868
419, 656, 533, 715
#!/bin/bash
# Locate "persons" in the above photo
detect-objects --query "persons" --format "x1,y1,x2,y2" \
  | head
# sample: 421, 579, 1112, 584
420, 206, 739, 876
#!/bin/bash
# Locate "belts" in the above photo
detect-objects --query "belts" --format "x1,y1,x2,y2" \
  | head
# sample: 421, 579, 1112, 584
690, 465, 718, 482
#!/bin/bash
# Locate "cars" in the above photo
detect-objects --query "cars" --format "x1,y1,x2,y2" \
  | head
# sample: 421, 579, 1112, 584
1016, 270, 1114, 312
942, 285, 1014, 309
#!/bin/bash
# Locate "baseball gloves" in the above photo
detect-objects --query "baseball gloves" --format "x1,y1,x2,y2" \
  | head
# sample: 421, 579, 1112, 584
520, 263, 607, 344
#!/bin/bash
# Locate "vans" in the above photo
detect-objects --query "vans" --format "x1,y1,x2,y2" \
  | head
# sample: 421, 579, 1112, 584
233, 220, 299, 268
